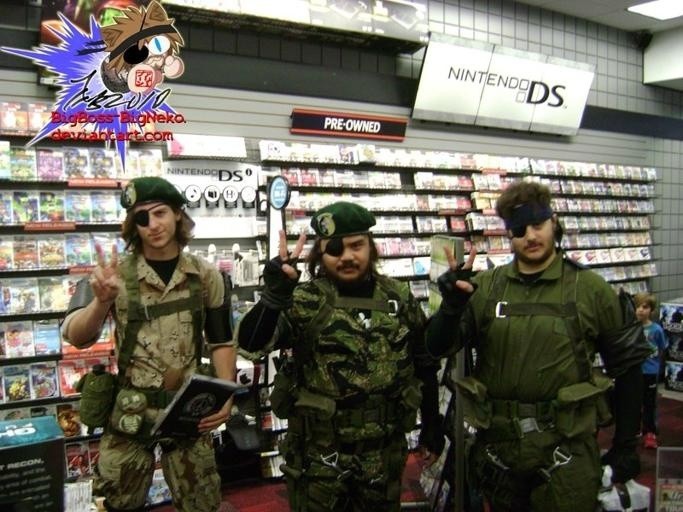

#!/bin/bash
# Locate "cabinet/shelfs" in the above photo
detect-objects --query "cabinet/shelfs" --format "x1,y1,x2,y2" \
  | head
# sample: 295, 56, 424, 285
0, 130, 660, 486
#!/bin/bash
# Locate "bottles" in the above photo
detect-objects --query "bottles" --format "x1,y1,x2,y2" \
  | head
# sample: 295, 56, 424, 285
77, 364, 120, 428
269, 354, 305, 421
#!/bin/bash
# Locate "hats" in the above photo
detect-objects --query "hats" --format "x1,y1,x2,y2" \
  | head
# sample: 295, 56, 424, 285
311, 203, 377, 236
121, 177, 188, 210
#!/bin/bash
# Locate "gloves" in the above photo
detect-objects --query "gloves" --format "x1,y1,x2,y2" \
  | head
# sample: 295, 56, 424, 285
601, 433, 641, 484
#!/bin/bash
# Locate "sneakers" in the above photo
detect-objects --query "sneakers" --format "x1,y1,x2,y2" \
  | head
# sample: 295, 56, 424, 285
643, 433, 658, 450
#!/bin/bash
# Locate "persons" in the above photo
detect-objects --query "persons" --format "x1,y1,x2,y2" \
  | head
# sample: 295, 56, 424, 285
0, 102, 682, 508
